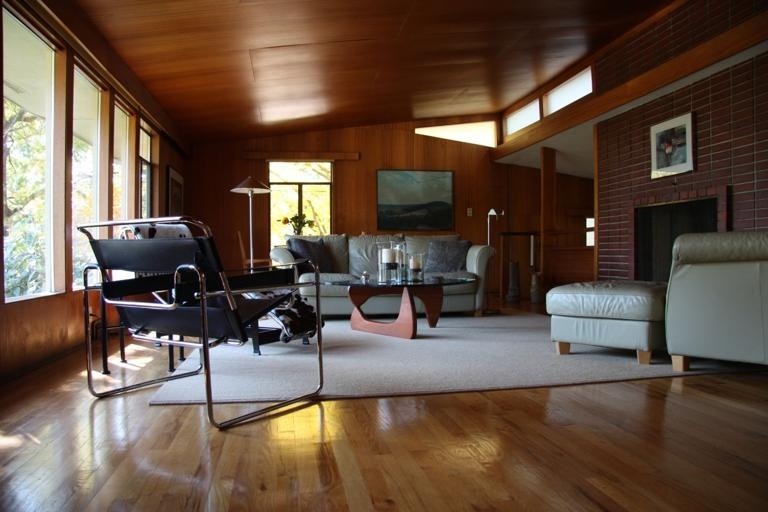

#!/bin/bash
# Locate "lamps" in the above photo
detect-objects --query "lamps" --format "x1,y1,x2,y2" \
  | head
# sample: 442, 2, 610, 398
482, 207, 505, 315
229, 175, 271, 274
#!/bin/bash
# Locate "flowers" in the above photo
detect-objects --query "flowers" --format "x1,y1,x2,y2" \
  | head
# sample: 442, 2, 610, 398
276, 213, 314, 229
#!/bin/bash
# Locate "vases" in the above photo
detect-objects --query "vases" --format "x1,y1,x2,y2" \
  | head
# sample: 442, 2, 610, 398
294, 229, 301, 235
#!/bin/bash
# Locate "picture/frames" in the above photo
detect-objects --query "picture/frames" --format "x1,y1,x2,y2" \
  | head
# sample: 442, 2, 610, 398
166, 165, 184, 216
650, 110, 697, 182
376, 169, 454, 232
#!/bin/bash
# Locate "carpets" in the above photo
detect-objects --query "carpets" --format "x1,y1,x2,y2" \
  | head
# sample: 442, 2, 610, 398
148, 307, 716, 405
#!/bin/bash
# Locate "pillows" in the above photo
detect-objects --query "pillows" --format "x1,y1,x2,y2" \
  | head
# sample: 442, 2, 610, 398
285, 233, 472, 275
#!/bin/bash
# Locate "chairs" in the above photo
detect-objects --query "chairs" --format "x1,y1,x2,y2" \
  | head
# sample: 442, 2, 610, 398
236, 229, 271, 274
663, 233, 768, 372
76, 215, 323, 429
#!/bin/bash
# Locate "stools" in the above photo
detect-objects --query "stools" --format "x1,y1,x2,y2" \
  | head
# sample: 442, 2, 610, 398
545, 279, 665, 365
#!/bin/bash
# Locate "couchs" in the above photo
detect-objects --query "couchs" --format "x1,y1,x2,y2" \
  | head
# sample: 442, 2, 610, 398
268, 244, 495, 318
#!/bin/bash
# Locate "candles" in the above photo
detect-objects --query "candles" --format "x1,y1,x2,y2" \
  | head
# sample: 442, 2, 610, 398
381, 248, 404, 265
410, 257, 420, 268
